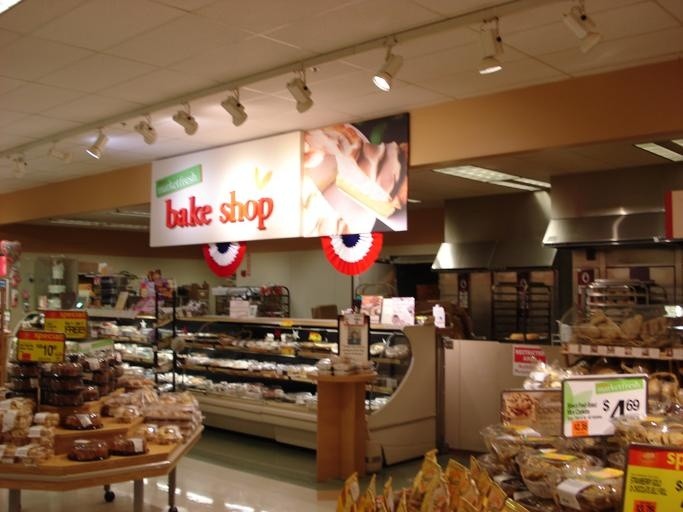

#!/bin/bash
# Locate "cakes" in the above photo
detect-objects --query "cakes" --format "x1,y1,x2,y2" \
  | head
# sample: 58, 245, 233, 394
302, 124, 408, 237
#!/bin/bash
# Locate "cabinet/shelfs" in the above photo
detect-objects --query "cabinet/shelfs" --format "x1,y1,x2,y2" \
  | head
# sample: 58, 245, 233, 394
432, 265, 573, 346
1, 294, 442, 511
566, 243, 682, 467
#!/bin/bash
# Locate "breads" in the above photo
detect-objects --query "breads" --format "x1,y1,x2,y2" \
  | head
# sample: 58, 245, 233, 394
484, 361, 683, 510
88, 318, 409, 413
573, 312, 673, 349
1, 353, 203, 467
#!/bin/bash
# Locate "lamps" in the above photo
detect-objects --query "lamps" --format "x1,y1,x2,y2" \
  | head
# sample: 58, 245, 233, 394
1, 0, 607, 183
